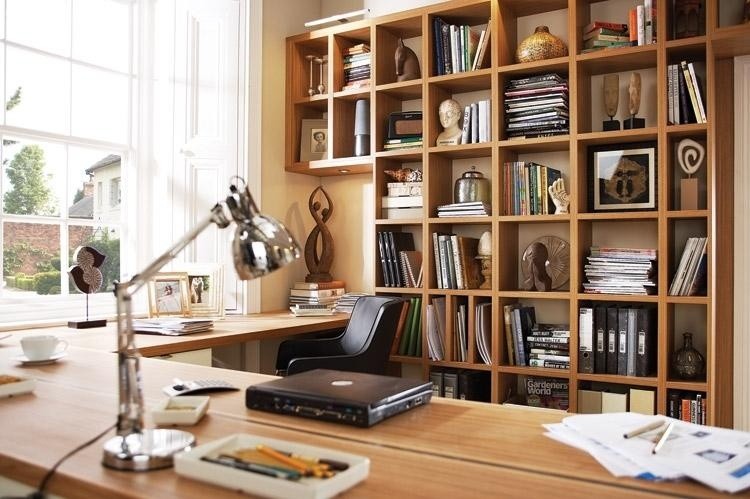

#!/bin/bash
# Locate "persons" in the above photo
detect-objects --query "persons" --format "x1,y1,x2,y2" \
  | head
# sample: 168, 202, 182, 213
436, 99, 463, 146
191, 277, 205, 303
313, 131, 328, 152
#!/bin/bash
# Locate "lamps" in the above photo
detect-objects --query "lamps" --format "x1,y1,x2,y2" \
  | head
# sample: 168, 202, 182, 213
98, 172, 306, 473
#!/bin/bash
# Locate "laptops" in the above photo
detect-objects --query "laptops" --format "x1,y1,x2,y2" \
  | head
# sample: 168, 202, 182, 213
246, 368, 433, 428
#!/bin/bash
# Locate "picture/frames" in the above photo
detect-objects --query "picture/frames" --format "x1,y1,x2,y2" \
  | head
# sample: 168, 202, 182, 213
179, 262, 226, 320
144, 271, 192, 319
299, 118, 329, 161
585, 142, 658, 210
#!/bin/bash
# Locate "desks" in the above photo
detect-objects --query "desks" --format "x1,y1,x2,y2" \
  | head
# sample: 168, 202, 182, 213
0, 306, 356, 372
0, 344, 750, 496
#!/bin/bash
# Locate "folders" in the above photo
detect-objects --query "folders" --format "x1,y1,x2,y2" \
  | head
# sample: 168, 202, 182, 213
578, 303, 602, 373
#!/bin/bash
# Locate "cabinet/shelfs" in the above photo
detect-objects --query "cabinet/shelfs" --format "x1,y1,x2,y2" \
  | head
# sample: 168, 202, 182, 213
373, 0, 733, 433
285, 17, 373, 178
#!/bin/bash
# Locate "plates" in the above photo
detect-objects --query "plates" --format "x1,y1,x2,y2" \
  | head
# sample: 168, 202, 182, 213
21, 359, 62, 366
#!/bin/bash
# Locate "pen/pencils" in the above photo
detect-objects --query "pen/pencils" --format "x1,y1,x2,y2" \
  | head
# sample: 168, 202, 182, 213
653, 421, 675, 454
200, 445, 349, 481
624, 420, 666, 438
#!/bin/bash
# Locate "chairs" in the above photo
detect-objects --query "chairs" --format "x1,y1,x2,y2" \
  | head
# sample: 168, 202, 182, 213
275, 293, 405, 382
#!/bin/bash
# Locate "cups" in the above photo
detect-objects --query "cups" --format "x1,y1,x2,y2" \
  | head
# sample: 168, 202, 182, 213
627, 71, 643, 114
20, 335, 70, 360
603, 72, 621, 120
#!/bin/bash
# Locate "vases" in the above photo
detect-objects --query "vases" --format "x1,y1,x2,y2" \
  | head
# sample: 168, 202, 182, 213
670, 330, 704, 382
514, 25, 568, 64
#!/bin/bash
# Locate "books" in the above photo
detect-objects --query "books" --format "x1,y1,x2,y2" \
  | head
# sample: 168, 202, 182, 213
384, 137, 423, 151
503, 162, 561, 216
503, 303, 569, 409
580, 0, 657, 54
437, 200, 492, 217
668, 238, 708, 297
128, 316, 215, 337
669, 393, 706, 425
433, 233, 485, 290
391, 297, 492, 365
288, 281, 369, 318
503, 73, 569, 141
582, 247, 658, 296
667, 61, 708, 126
378, 232, 423, 288
342, 44, 372, 91
432, 17, 492, 75
460, 99, 491, 144
